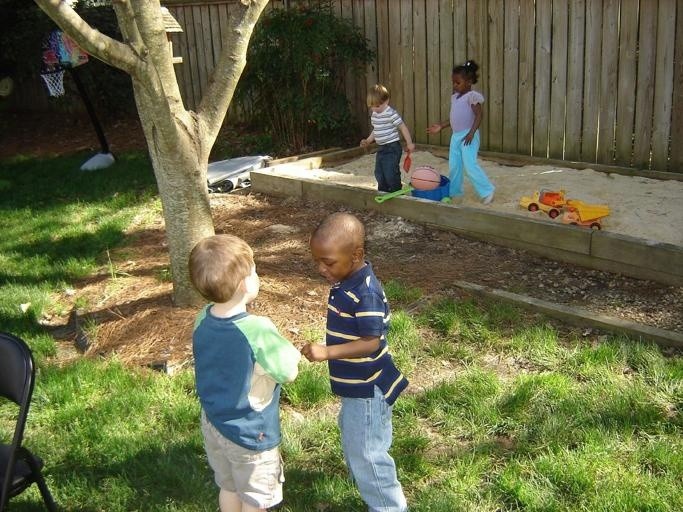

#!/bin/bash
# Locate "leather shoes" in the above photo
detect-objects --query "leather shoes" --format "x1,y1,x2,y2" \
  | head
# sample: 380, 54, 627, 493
484, 191, 494, 205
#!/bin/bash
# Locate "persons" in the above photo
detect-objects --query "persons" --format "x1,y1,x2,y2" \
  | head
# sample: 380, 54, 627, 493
357, 82, 416, 196
421, 58, 498, 207
297, 209, 411, 512
183, 231, 304, 512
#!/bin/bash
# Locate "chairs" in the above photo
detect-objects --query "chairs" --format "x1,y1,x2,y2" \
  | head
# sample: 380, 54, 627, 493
1, 331, 60, 512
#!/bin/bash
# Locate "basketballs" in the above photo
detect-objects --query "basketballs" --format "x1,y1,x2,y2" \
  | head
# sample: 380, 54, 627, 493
411, 165, 441, 190
